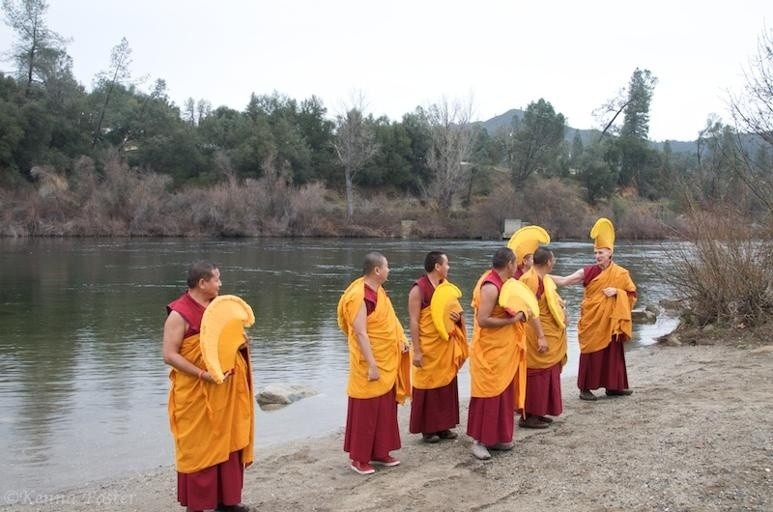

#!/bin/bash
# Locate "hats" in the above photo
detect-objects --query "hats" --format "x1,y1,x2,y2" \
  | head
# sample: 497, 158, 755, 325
499, 275, 539, 322
590, 217, 615, 251
506, 225, 551, 265
543, 274, 567, 329
430, 283, 464, 342
199, 295, 255, 384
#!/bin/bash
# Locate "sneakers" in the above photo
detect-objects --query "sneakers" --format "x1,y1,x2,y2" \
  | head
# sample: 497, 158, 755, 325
580, 393, 598, 401
215, 503, 249, 512
605, 389, 634, 397
368, 453, 401, 469
470, 442, 493, 460
491, 442, 514, 452
351, 460, 376, 476
422, 430, 458, 443
518, 414, 553, 429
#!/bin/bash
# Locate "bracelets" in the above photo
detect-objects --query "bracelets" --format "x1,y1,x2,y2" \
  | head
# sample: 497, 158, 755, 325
198, 370, 204, 381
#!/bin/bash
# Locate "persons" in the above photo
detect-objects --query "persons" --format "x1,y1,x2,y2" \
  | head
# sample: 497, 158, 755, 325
406, 251, 469, 443
545, 218, 636, 401
161, 261, 254, 512
335, 251, 412, 475
466, 247, 531, 461
506, 224, 549, 280
516, 245, 567, 429
